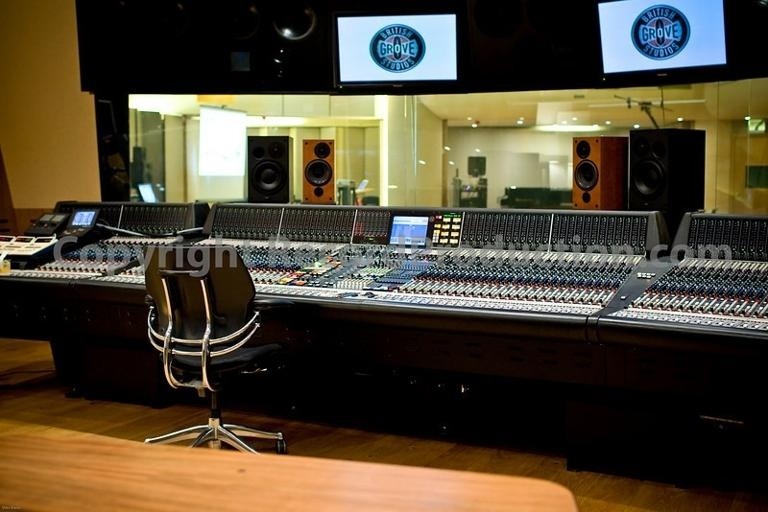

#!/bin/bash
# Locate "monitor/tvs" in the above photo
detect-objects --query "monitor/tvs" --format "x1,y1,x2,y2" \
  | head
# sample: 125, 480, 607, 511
137, 183, 157, 203
390, 216, 429, 246
332, 10, 459, 90
597, 0, 729, 82
72, 211, 96, 226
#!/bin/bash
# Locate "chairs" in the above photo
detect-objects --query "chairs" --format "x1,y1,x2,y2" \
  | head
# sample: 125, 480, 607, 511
141, 242, 297, 455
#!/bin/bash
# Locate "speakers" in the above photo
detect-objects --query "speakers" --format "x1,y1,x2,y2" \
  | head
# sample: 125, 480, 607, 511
629, 129, 705, 210
247, 136, 294, 204
572, 136, 629, 210
303, 139, 335, 205
